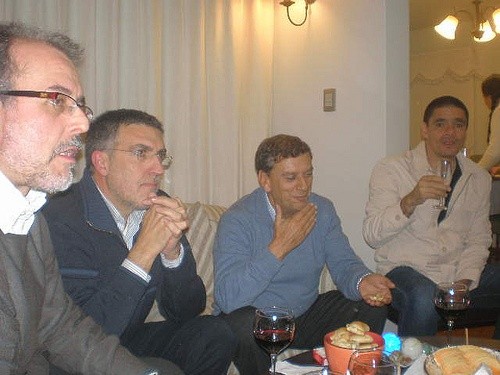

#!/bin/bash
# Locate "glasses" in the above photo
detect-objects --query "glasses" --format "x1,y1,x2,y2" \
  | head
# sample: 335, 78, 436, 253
113, 148, 173, 170
0, 91, 94, 122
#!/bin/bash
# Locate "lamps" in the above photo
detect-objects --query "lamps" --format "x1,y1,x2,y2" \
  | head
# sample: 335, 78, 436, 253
277, 0, 320, 26
432, 0, 500, 49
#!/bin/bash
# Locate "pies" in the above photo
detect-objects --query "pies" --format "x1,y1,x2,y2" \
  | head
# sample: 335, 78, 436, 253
425, 344, 500, 375
330, 321, 379, 349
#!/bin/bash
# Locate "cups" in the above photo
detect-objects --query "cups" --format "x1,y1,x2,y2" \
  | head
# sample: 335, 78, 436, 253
347, 348, 398, 375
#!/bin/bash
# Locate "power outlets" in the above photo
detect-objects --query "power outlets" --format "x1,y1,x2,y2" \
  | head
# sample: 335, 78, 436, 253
324, 89, 337, 113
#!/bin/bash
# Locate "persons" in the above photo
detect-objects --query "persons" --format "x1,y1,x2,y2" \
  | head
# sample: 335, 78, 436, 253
0, 21, 162, 375
37, 108, 239, 374
474, 74, 500, 175
214, 135, 396, 375
362, 96, 500, 342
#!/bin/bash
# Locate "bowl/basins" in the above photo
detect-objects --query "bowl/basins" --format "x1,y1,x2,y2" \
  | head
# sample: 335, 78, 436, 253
323, 330, 384, 375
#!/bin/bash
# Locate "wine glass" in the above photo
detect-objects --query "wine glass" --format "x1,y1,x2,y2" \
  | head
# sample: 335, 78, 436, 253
433, 159, 452, 210
252, 306, 295, 375
434, 283, 472, 347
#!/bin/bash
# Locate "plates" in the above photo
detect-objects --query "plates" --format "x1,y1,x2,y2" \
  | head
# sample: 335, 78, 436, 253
423, 345, 500, 375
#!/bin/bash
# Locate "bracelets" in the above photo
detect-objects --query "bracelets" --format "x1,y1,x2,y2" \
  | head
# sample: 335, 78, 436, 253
175, 243, 181, 259
357, 272, 373, 292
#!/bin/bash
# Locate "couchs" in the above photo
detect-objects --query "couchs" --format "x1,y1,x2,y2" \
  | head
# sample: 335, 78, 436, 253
144, 205, 336, 324
